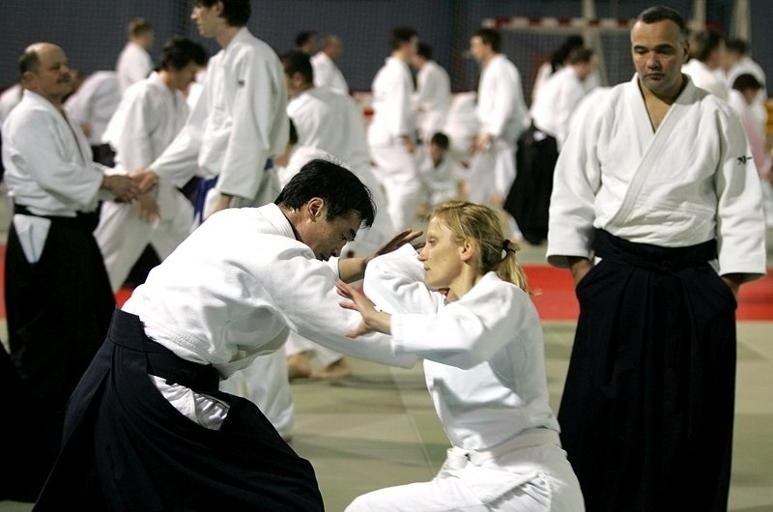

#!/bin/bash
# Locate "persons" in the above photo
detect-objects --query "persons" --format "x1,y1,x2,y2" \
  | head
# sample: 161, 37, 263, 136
0, 1, 773, 512
